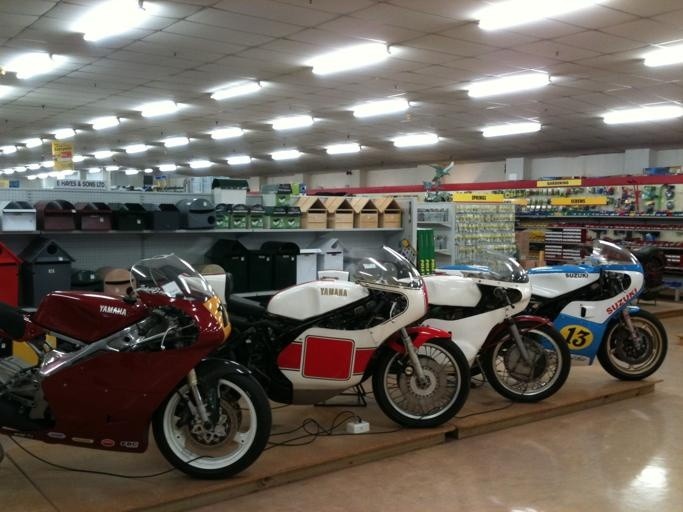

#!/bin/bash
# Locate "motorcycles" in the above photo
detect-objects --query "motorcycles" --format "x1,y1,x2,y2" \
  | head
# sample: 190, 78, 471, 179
200, 244, 472, 429
370, 250, 571, 404
0, 249, 272, 482
428, 238, 669, 384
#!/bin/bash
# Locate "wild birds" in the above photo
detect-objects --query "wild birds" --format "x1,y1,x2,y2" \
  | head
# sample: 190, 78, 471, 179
428, 160, 454, 182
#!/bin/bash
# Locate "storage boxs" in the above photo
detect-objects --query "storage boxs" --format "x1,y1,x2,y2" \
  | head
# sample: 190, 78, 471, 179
294, 195, 403, 230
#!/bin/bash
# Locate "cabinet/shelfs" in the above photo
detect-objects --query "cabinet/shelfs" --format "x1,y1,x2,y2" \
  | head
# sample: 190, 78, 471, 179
0, 188, 417, 312
415, 175, 683, 303
